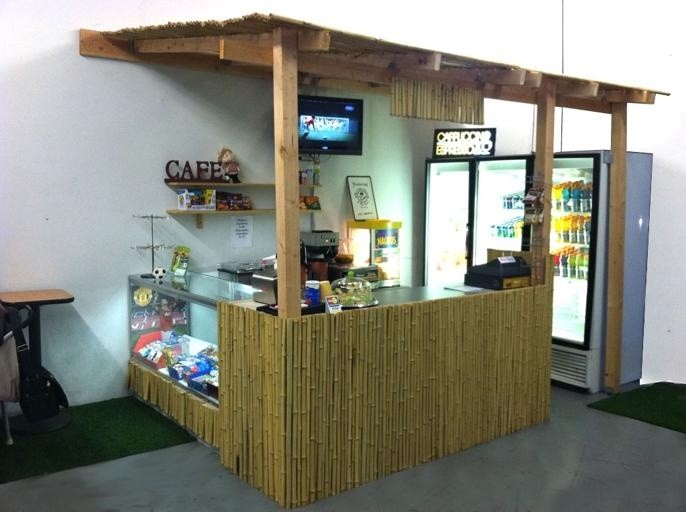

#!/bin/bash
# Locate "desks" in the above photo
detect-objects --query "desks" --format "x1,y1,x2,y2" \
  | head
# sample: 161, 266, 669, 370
0, 287, 73, 435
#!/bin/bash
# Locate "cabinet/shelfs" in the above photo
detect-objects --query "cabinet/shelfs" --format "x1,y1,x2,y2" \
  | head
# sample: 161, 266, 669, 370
488, 207, 592, 287
165, 179, 325, 216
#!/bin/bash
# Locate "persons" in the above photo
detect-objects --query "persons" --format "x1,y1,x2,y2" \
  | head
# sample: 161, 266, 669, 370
217, 147, 245, 183
301, 115, 316, 139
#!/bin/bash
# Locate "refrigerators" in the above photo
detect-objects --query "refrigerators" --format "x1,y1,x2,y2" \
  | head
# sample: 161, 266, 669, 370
472, 153, 533, 279
423, 157, 472, 287
525, 148, 653, 395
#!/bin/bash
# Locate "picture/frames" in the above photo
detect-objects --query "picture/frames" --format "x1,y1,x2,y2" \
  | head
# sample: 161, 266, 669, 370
345, 175, 380, 221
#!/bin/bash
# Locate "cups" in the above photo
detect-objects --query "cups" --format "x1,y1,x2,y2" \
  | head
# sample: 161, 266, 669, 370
305, 280, 320, 306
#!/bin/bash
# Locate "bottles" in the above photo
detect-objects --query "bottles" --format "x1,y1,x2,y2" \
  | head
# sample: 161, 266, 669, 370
550, 177, 592, 277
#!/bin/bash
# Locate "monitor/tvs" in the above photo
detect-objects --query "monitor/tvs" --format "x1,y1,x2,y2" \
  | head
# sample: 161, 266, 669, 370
298, 95, 363, 155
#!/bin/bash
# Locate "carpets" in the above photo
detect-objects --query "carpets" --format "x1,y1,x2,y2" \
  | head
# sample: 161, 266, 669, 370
0, 394, 199, 485
585, 380, 686, 434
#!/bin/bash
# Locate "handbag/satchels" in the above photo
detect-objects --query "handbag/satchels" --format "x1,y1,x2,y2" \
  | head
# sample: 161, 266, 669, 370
8, 317, 69, 424
